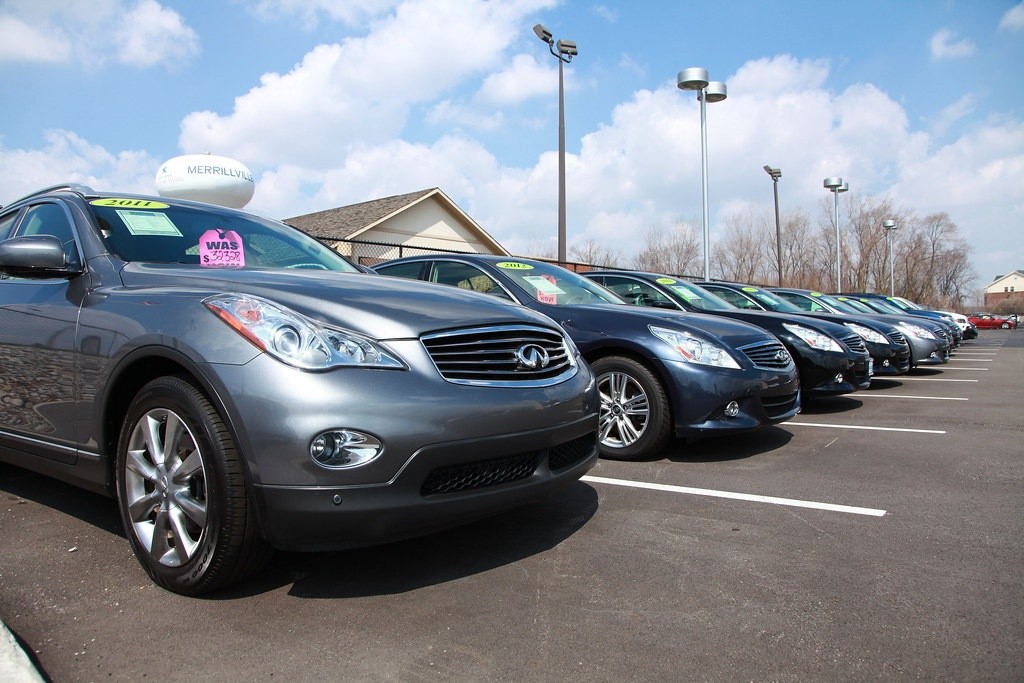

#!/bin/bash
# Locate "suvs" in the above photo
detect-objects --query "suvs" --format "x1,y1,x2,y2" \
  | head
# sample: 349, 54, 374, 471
0, 182, 602, 599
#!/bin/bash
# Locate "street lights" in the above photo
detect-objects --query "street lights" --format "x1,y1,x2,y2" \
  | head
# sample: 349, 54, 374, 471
764, 165, 783, 289
883, 219, 898, 297
824, 177, 848, 294
677, 67, 728, 284
534, 23, 579, 262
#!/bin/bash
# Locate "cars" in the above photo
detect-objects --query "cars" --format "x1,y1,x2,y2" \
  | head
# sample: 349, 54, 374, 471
369, 253, 802, 462
759, 286, 979, 374
967, 312, 1015, 330
1008, 313, 1021, 323
570, 269, 874, 399
623, 281, 911, 378
